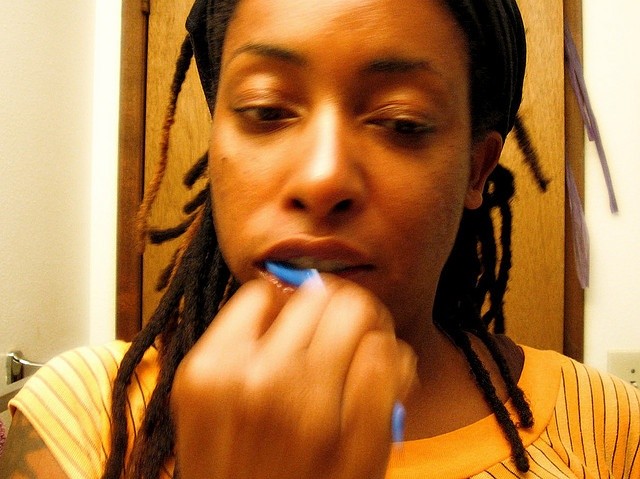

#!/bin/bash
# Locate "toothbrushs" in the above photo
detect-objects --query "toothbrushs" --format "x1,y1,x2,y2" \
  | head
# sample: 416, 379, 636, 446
260, 256, 406, 442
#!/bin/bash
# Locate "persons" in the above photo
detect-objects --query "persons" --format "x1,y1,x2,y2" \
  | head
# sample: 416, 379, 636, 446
0, 1, 640, 479
